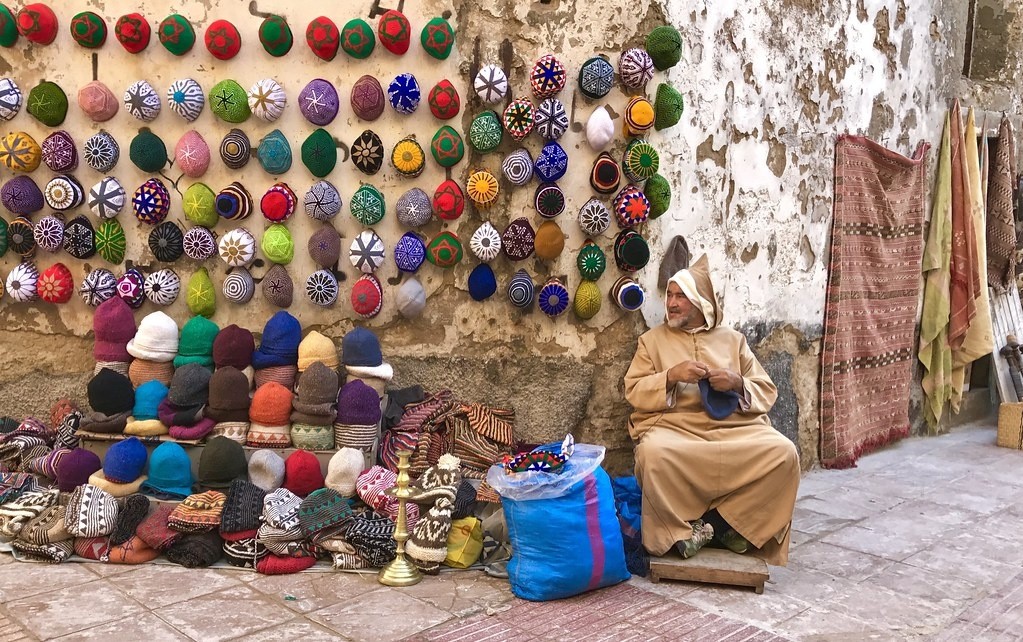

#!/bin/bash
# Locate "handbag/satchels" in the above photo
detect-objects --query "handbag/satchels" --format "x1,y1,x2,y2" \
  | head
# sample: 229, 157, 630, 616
441, 516, 483, 568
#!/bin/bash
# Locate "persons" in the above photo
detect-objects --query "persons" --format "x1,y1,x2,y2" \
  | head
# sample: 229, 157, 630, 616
623, 255, 800, 560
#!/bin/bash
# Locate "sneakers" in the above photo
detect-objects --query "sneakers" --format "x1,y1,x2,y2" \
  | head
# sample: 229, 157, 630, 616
676, 519, 713, 558
716, 530, 756, 554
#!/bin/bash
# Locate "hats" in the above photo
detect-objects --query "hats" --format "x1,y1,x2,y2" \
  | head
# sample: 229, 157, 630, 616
647, 26, 682, 72
501, 433, 576, 472
0, 3, 569, 318
654, 83, 684, 131
589, 151, 620, 195
613, 230, 651, 271
609, 278, 646, 312
657, 237, 689, 290
698, 376, 744, 419
573, 280, 603, 320
576, 239, 606, 281
621, 49, 656, 88
586, 106, 614, 151
578, 57, 614, 97
0, 296, 499, 575
644, 172, 672, 218
625, 96, 656, 135
622, 139, 658, 181
609, 185, 650, 229
579, 198, 609, 237
